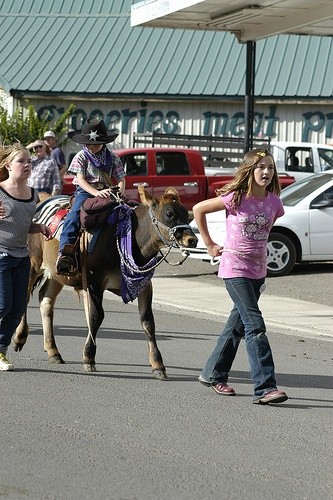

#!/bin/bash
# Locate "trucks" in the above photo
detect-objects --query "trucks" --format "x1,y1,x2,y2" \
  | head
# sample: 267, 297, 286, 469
132, 132, 333, 182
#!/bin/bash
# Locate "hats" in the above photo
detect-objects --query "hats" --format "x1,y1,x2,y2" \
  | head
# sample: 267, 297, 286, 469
42, 131, 55, 138
67, 119, 119, 145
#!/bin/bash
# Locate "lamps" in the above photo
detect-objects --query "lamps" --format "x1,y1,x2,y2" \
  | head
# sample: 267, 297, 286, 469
197, 4, 275, 28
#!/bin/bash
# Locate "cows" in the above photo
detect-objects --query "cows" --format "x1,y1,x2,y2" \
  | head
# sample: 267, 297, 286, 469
12, 183, 199, 382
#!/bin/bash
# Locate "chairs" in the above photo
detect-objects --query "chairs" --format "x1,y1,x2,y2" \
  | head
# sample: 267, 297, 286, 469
301, 157, 312, 172
288, 156, 300, 171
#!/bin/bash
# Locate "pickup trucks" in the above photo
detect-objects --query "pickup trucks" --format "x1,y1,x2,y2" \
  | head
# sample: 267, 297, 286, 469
62, 149, 295, 212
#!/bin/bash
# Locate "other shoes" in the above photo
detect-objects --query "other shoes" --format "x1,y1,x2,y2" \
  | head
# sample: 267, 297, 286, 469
0, 353, 14, 370
55, 255, 72, 272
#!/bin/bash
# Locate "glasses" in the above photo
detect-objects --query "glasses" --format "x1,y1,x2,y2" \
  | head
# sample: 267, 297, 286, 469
35, 146, 42, 148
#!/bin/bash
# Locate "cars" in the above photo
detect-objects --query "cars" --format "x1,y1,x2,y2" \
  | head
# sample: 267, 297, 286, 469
182, 170, 333, 277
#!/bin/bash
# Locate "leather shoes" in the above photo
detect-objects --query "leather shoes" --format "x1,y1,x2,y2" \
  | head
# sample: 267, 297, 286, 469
253, 390, 288, 404
199, 380, 235, 395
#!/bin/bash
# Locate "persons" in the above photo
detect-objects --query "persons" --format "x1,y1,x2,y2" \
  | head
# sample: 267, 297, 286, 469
26, 140, 61, 196
0, 136, 52, 372
193, 148, 288, 404
42, 130, 67, 175
55, 118, 127, 276
